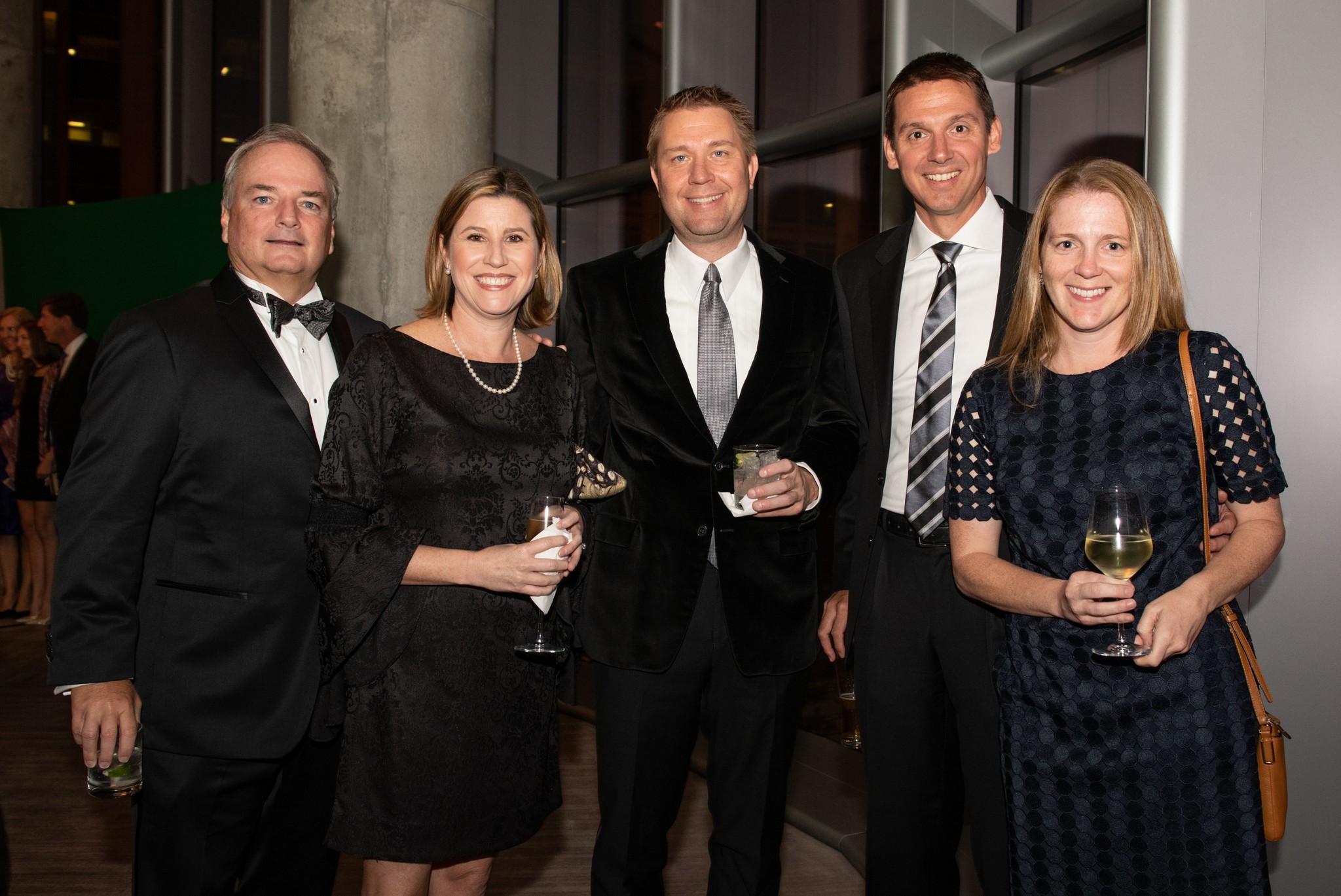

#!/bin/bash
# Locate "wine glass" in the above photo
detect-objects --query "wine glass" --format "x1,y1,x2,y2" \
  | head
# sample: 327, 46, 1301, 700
1084, 483, 1154, 659
515, 494, 568, 655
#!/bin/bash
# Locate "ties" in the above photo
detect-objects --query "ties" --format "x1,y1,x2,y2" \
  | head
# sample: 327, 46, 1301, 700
697, 263, 738, 449
905, 240, 963, 541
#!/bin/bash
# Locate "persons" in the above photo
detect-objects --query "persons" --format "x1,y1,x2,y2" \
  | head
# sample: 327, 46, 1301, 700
1, 290, 93, 627
41, 121, 370, 895
292, 162, 596, 896
945, 154, 1290, 896
550, 86, 862, 896
810, 46, 1239, 895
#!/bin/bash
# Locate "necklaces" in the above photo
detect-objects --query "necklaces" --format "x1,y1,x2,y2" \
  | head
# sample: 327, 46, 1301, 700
440, 312, 522, 394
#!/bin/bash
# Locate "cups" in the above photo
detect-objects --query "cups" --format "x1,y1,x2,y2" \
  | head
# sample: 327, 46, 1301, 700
732, 443, 781, 510
86, 720, 143, 798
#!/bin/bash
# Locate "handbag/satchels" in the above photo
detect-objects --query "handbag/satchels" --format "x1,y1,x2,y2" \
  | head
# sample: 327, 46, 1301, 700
1256, 711, 1292, 842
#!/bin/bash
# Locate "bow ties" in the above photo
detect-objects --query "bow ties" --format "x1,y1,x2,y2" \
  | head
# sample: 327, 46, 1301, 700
232, 267, 336, 340
